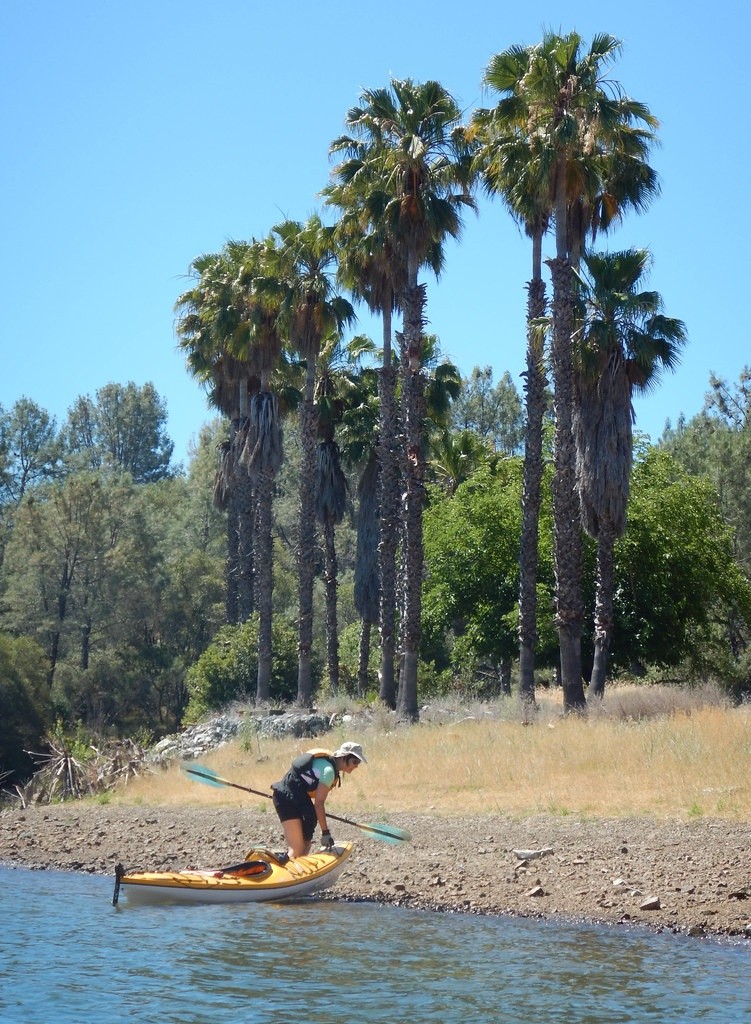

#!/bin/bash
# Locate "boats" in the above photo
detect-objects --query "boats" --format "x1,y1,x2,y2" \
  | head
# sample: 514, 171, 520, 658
109, 840, 358, 907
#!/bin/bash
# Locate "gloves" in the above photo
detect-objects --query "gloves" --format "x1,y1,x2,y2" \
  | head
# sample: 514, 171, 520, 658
321, 829, 334, 849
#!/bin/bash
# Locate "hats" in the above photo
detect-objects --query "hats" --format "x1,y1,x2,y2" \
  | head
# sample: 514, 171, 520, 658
340, 742, 368, 763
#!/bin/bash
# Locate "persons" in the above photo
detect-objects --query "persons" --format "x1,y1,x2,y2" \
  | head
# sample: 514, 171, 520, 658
268, 741, 369, 859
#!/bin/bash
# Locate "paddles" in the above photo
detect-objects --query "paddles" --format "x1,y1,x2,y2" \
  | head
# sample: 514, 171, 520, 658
179, 761, 412, 848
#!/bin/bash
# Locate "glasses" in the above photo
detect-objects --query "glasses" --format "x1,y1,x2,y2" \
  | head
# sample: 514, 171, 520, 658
353, 759, 360, 765
